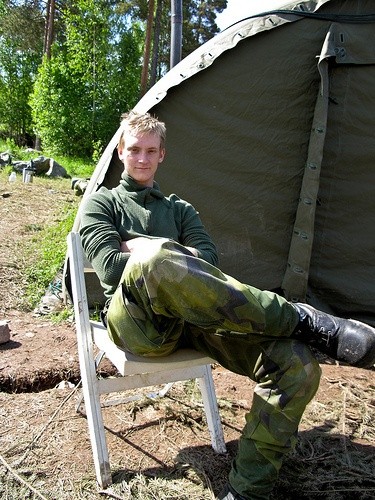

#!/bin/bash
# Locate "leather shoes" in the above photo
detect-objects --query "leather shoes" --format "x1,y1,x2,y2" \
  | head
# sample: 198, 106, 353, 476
215, 479, 246, 500
293, 302, 375, 369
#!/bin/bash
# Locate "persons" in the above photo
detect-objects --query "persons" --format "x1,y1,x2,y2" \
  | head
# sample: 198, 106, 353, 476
76, 110, 375, 499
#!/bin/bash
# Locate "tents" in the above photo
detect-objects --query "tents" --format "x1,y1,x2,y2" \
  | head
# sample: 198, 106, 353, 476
64, 0, 375, 328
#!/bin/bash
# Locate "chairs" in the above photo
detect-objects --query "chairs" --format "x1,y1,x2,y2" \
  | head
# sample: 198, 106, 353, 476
62, 231, 228, 489
